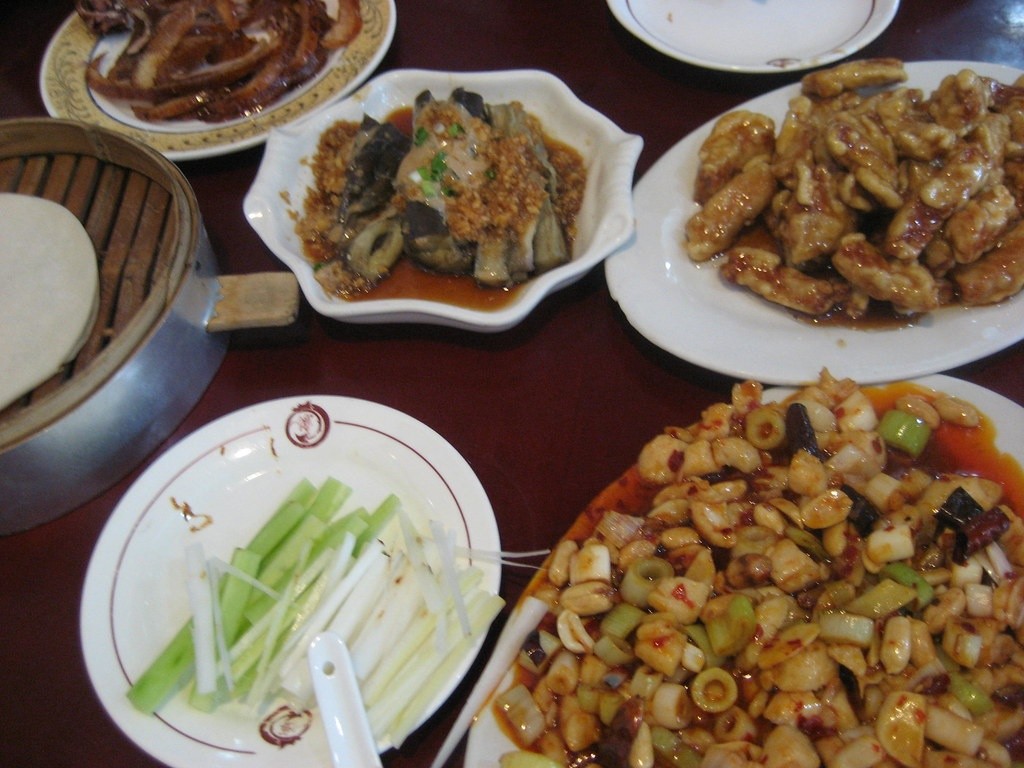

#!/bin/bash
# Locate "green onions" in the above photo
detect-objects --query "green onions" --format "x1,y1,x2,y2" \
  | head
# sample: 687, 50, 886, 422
496, 394, 1024, 768
410, 121, 497, 197
126, 477, 550, 768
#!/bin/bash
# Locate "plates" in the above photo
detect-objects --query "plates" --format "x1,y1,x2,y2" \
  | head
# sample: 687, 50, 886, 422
605, 0, 901, 73
79, 396, 502, 768
463, 375, 1024, 766
606, 60, 1024, 388
241, 66, 645, 333
38, 0, 397, 161
